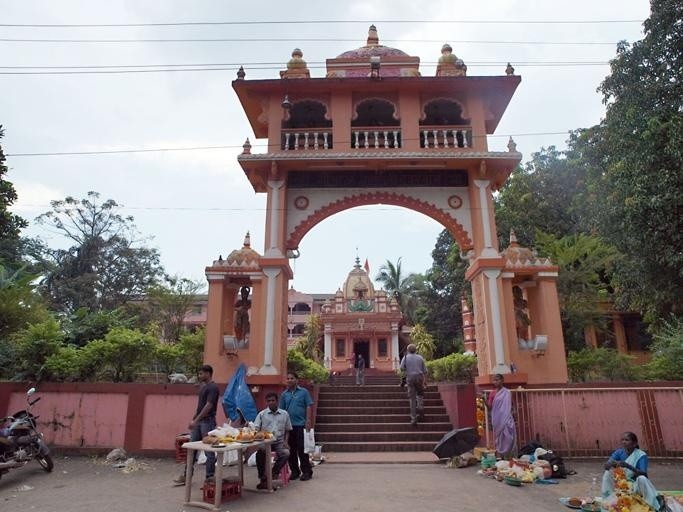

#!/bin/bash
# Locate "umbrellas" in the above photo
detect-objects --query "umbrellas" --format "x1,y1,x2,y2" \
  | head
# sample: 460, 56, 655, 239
431, 426, 482, 459
222, 363, 258, 427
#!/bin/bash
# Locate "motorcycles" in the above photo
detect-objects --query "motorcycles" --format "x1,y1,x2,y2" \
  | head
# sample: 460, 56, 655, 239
0, 387, 54, 482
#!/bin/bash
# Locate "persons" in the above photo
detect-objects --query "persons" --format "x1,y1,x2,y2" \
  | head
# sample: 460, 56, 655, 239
399, 343, 428, 426
594, 429, 661, 511
355, 354, 365, 387
249, 392, 294, 491
231, 285, 251, 344
512, 287, 534, 341
169, 362, 220, 489
276, 371, 313, 481
476, 372, 516, 460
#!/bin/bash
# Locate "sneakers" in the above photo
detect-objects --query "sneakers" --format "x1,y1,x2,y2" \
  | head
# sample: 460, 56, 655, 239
299, 471, 312, 480
417, 407, 424, 417
172, 475, 186, 483
289, 471, 301, 480
410, 418, 418, 423
272, 473, 279, 481
257, 478, 267, 489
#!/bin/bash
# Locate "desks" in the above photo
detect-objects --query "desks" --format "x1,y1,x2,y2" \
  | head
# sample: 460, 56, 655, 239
180, 438, 276, 511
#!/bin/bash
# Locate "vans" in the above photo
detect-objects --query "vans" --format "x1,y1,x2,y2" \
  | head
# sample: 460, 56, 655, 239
168, 373, 188, 383
